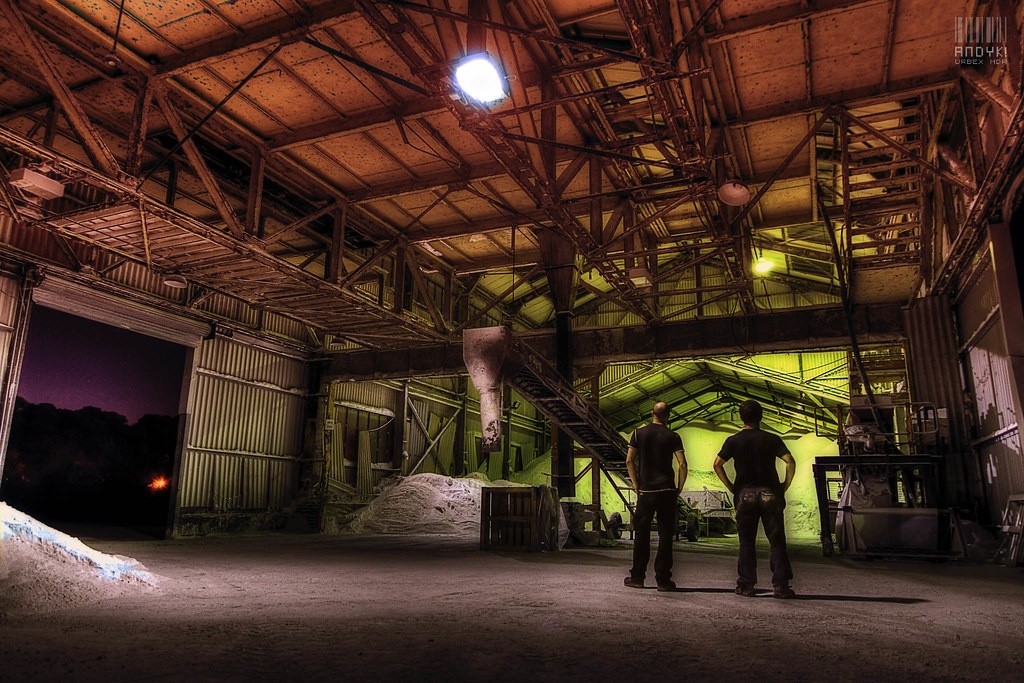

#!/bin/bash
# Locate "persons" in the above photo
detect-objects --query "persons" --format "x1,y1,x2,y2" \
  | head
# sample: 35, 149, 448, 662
624, 402, 687, 591
713, 400, 796, 599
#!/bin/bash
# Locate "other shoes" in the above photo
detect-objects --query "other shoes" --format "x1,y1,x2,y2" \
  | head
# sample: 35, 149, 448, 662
774, 589, 796, 599
735, 585, 755, 597
624, 576, 644, 588
657, 581, 676, 591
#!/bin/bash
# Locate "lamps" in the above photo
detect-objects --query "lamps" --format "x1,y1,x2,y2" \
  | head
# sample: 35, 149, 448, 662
719, 179, 751, 207
455, 52, 509, 104
